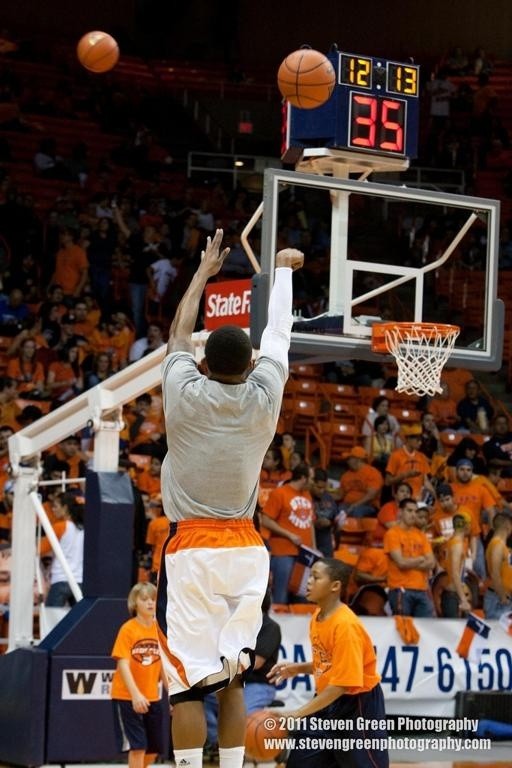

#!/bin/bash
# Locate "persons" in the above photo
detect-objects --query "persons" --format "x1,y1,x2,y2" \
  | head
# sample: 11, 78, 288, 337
0, 42, 512, 768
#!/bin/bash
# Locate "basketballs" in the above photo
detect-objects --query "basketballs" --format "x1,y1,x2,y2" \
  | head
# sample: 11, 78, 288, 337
278, 49, 336, 107
244, 709, 288, 760
78, 31, 119, 72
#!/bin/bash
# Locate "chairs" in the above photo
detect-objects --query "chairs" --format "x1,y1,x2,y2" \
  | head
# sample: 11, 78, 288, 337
267, 270, 511, 616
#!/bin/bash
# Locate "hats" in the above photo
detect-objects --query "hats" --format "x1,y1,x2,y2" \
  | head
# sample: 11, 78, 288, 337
416, 500, 435, 517
342, 446, 367, 460
